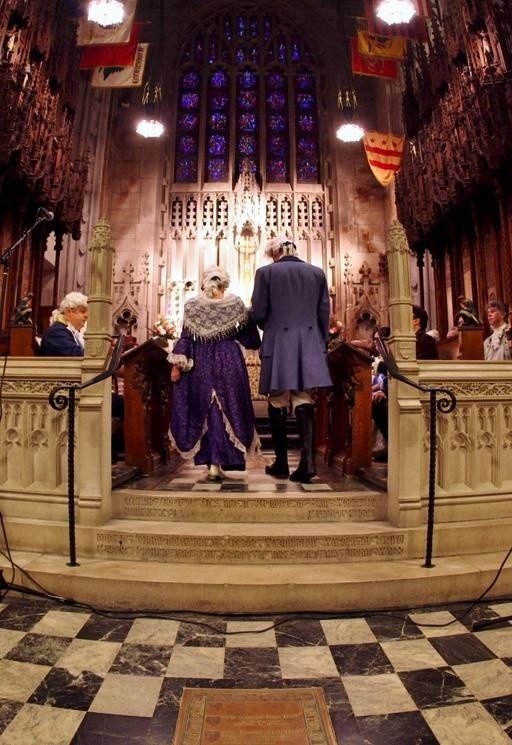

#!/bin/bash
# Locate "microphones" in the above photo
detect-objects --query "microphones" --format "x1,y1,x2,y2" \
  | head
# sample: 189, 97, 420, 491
39, 207, 54, 221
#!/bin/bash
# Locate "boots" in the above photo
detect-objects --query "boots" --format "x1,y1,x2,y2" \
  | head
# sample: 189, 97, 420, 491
264, 401, 288, 480
289, 403, 317, 480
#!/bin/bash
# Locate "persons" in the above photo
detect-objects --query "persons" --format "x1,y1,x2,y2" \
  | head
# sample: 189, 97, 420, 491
14, 291, 36, 325
247, 233, 336, 481
327, 321, 346, 338
370, 327, 391, 394
40, 290, 124, 460
444, 314, 478, 360
165, 265, 260, 482
372, 304, 438, 464
483, 301, 508, 361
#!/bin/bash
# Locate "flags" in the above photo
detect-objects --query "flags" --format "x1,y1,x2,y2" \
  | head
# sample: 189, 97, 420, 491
375, 0, 432, 20
361, 130, 408, 186
76, 0, 137, 44
365, 0, 429, 43
80, 22, 145, 70
350, 36, 399, 80
355, 16, 409, 60
91, 42, 149, 88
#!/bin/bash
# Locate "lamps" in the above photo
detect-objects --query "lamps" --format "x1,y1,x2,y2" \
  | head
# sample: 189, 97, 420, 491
131, 0, 170, 145
328, 3, 368, 145
84, 0, 128, 31
372, 1, 417, 31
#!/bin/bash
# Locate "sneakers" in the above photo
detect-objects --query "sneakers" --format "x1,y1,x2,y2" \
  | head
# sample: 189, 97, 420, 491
372, 446, 388, 462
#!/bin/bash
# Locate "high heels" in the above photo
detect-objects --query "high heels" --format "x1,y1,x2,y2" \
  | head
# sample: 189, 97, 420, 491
208, 464, 226, 480
